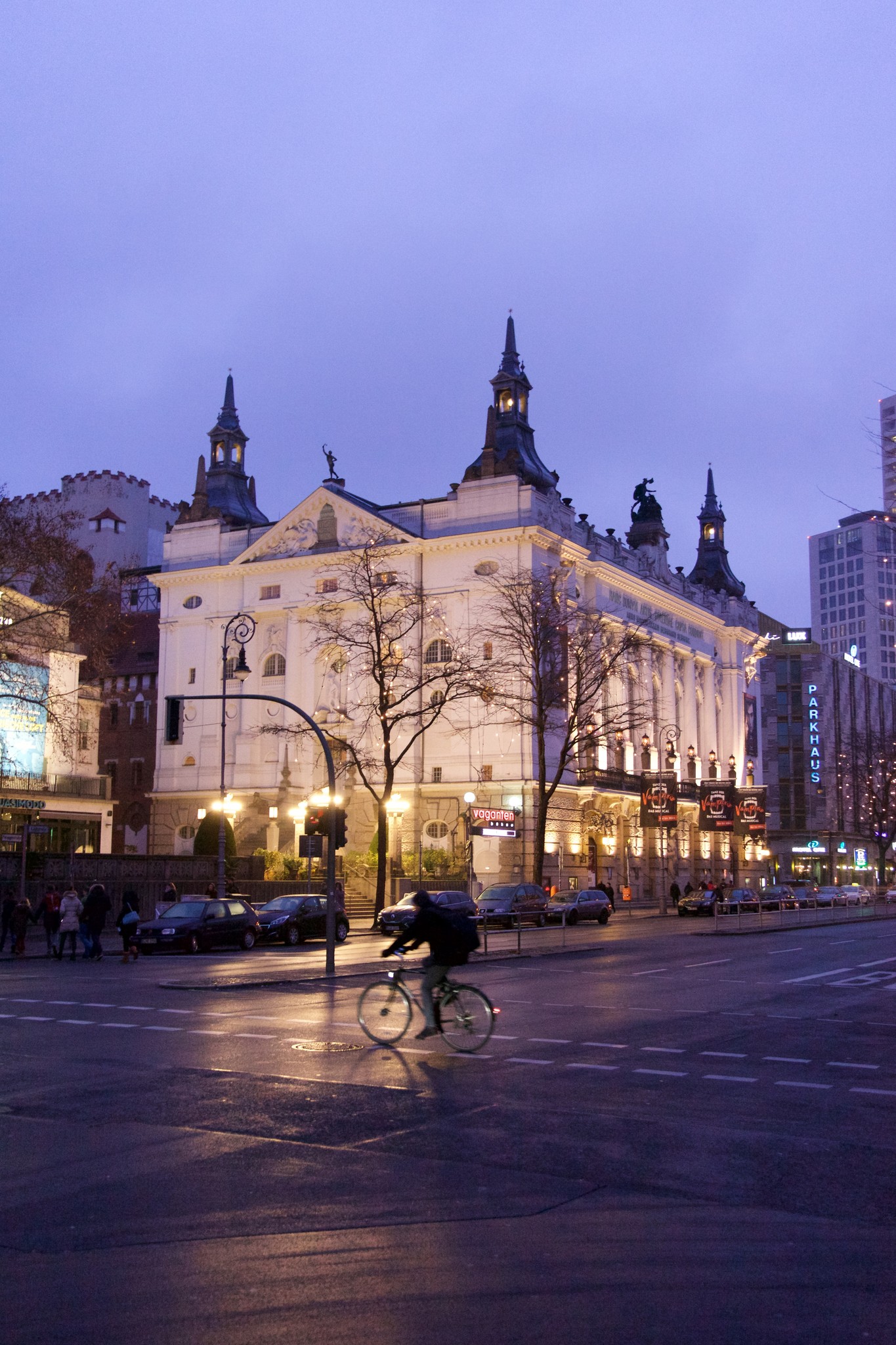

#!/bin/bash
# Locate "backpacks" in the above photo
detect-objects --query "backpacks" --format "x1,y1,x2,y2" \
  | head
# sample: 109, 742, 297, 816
419, 903, 481, 957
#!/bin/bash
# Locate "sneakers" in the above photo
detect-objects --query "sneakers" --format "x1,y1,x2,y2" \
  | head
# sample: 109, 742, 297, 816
95, 954, 104, 961
414, 1025, 439, 1040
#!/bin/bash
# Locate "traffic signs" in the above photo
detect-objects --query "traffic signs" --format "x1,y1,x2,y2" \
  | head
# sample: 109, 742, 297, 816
28, 825, 48, 834
1, 833, 23, 843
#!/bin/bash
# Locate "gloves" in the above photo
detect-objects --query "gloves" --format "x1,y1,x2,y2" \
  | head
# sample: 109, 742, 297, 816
380, 944, 400, 957
398, 942, 418, 953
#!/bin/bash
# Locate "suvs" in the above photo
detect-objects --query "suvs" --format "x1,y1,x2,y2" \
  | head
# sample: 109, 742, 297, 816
544, 889, 612, 926
781, 879, 819, 908
474, 882, 548, 927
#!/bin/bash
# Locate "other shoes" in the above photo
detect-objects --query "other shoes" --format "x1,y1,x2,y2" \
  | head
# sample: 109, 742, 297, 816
45, 948, 52, 955
51, 942, 58, 955
69, 952, 76, 961
56, 950, 62, 960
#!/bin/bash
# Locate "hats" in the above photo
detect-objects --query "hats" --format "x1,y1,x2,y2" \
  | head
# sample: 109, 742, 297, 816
410, 889, 432, 906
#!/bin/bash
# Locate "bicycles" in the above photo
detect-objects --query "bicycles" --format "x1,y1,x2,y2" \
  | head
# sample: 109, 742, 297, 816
357, 948, 504, 1053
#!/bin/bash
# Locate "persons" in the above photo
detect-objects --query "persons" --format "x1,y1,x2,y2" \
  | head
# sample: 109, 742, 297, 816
79, 887, 95, 962
55, 883, 84, 963
334, 881, 345, 909
225, 877, 241, 893
595, 879, 606, 892
81, 879, 112, 961
35, 884, 63, 956
320, 883, 328, 895
115, 883, 141, 964
380, 889, 468, 1039
10, 896, 38, 956
161, 881, 177, 902
876, 877, 895, 901
670, 878, 736, 912
0, 888, 19, 954
204, 883, 218, 898
786, 872, 822, 887
604, 882, 615, 912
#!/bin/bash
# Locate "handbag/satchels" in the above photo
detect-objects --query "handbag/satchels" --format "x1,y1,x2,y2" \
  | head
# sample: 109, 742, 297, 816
120, 913, 142, 926
76, 921, 95, 953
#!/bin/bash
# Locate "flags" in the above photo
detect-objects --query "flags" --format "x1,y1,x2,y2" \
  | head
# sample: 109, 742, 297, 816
734, 787, 766, 838
639, 772, 677, 828
698, 779, 735, 832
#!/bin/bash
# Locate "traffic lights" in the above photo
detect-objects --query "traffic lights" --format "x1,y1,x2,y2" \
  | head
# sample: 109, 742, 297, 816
316, 806, 329, 837
335, 808, 348, 850
305, 806, 318, 835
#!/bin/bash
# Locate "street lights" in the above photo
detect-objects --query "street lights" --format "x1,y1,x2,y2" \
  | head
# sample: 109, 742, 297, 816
163, 691, 336, 974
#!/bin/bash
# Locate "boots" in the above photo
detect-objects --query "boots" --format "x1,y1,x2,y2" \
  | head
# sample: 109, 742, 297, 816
119, 951, 130, 965
128, 946, 139, 960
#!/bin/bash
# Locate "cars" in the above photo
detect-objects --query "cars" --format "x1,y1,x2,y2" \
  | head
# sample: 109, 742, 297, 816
813, 886, 849, 907
720, 888, 761, 913
377, 890, 479, 935
255, 894, 350, 946
840, 882, 870, 906
134, 898, 260, 956
678, 889, 722, 918
886, 885, 896, 902
754, 883, 797, 912
